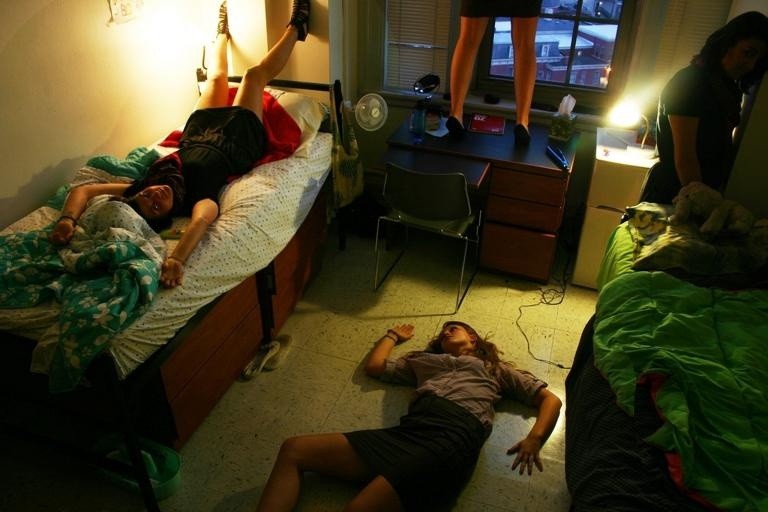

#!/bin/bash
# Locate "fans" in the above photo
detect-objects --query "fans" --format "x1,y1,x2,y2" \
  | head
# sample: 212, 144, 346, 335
345, 92, 386, 133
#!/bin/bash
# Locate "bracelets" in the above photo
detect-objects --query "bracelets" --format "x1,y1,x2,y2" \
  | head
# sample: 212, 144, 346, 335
57, 214, 77, 223
379, 328, 401, 343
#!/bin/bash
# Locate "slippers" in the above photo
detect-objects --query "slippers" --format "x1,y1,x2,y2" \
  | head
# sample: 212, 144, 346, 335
264, 334, 293, 370
241, 340, 280, 379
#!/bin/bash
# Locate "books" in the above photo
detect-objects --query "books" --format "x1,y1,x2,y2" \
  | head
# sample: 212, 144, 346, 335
469, 111, 506, 135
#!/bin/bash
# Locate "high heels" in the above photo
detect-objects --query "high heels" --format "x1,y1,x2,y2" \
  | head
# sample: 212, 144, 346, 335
513, 122, 530, 149
444, 114, 468, 139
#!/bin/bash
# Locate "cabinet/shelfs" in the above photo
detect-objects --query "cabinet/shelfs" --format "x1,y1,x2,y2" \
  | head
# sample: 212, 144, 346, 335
571, 143, 659, 291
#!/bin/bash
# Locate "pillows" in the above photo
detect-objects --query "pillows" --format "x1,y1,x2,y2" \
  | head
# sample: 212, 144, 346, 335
192, 83, 327, 157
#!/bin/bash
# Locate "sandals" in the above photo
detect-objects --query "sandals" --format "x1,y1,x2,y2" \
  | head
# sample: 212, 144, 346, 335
215, 1, 232, 35
284, 2, 311, 40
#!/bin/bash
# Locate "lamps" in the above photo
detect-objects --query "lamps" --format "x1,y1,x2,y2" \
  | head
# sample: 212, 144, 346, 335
604, 97, 649, 148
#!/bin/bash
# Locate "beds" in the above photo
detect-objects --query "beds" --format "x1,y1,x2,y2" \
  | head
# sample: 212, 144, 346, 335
0, 69, 340, 450
589, 202, 763, 512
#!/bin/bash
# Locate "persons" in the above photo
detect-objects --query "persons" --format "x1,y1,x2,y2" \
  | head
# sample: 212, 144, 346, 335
49, 0, 312, 288
256, 322, 564, 511
639, 10, 768, 208
444, 0, 542, 149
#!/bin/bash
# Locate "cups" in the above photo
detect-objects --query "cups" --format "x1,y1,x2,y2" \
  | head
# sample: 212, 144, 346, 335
411, 109, 425, 144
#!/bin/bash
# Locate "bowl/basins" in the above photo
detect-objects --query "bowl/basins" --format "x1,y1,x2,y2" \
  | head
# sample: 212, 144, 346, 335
137, 445, 186, 501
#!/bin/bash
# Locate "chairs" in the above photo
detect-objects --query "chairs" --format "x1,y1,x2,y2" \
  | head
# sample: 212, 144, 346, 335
369, 162, 483, 313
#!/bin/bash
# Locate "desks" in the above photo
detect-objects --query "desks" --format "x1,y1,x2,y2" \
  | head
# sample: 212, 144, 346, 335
385, 113, 580, 294
